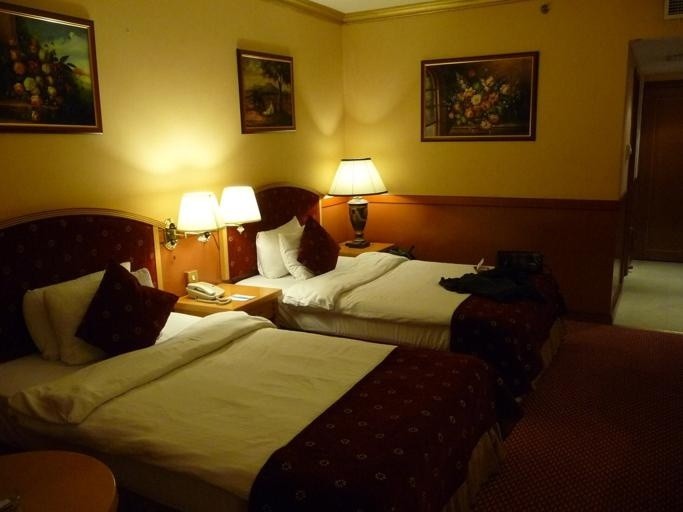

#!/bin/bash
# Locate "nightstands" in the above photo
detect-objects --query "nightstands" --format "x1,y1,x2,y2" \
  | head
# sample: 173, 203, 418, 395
173, 282, 282, 328
335, 241, 396, 258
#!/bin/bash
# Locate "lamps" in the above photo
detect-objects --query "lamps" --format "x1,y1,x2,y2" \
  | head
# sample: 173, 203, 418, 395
326, 158, 389, 249
220, 186, 262, 226
177, 191, 225, 235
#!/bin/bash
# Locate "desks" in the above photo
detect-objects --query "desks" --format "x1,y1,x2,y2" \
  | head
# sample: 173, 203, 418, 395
0, 451, 119, 512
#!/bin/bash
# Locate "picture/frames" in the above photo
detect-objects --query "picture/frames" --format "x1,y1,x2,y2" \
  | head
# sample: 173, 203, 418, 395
0, 2, 104, 135
237, 47, 296, 133
421, 50, 539, 142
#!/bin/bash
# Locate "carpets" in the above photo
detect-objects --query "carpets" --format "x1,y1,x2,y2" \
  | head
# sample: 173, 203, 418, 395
466, 319, 683, 512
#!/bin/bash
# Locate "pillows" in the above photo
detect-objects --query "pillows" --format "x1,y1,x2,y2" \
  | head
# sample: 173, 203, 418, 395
45, 267, 155, 366
22, 262, 130, 362
75, 259, 179, 355
280, 232, 319, 282
254, 215, 304, 279
297, 215, 341, 272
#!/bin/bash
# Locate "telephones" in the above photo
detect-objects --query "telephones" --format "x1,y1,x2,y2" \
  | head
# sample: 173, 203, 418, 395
185, 282, 227, 301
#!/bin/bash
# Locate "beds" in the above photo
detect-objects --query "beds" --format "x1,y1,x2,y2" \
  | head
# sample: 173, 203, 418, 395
0, 208, 505, 512
228, 181, 567, 398
